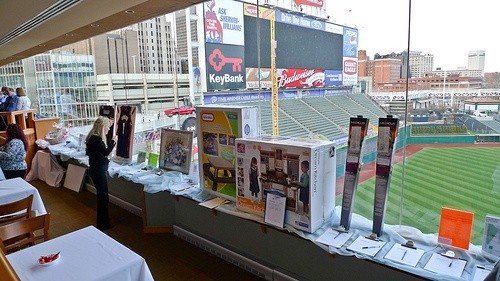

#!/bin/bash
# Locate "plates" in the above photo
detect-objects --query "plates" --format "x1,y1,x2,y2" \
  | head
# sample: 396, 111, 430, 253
35, 252, 61, 267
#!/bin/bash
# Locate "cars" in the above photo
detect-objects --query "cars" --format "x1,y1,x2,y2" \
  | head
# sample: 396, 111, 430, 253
164, 105, 196, 118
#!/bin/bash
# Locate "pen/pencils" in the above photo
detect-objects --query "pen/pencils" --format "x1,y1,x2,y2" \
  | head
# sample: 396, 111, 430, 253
448, 257, 454, 269
362, 244, 380, 251
475, 264, 492, 273
400, 249, 408, 263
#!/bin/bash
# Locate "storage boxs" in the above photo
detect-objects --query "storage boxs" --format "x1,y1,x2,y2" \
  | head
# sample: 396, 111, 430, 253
63, 164, 88, 193
235, 134, 337, 232
194, 104, 260, 202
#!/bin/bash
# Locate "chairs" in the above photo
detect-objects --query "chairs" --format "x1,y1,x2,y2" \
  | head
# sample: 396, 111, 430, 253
0, 212, 51, 255
0, 194, 33, 226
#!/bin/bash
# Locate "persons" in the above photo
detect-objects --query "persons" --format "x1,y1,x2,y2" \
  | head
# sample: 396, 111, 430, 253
236, 143, 246, 199
39, 87, 78, 119
86, 116, 118, 231
0, 123, 27, 180
0, 86, 31, 131
248, 157, 260, 200
291, 160, 309, 218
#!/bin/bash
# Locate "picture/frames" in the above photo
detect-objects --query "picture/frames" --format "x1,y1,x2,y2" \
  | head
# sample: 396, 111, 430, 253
182, 117, 197, 138
158, 127, 193, 176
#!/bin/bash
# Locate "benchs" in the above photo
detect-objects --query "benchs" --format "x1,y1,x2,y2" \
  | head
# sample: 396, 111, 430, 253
226, 93, 387, 141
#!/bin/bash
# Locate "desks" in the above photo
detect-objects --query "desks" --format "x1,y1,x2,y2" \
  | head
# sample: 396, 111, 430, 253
0, 177, 47, 216
5, 225, 154, 281
